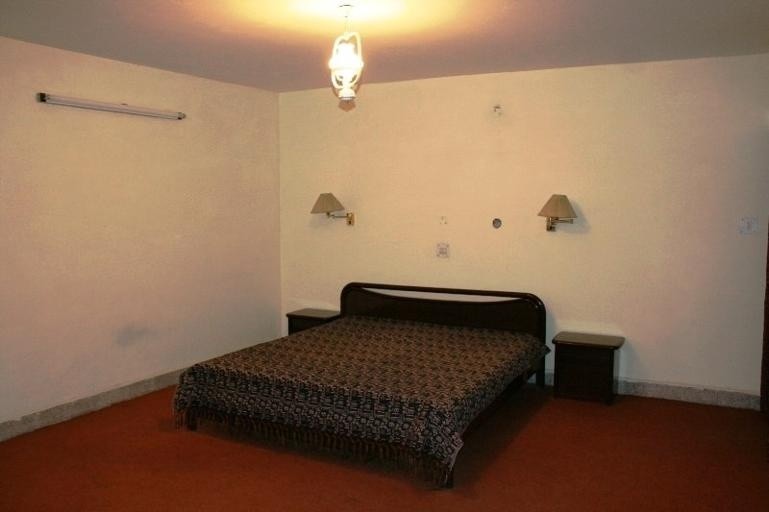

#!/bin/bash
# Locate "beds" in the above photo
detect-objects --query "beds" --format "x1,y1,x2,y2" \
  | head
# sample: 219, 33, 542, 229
174, 282, 552, 489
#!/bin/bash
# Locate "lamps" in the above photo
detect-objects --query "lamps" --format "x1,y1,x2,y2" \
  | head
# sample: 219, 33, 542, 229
536, 193, 577, 230
309, 192, 355, 226
326, 0, 369, 109
37, 92, 185, 121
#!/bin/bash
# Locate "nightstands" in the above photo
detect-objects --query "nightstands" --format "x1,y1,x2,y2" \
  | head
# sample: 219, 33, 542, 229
551, 331, 625, 405
285, 307, 342, 337
